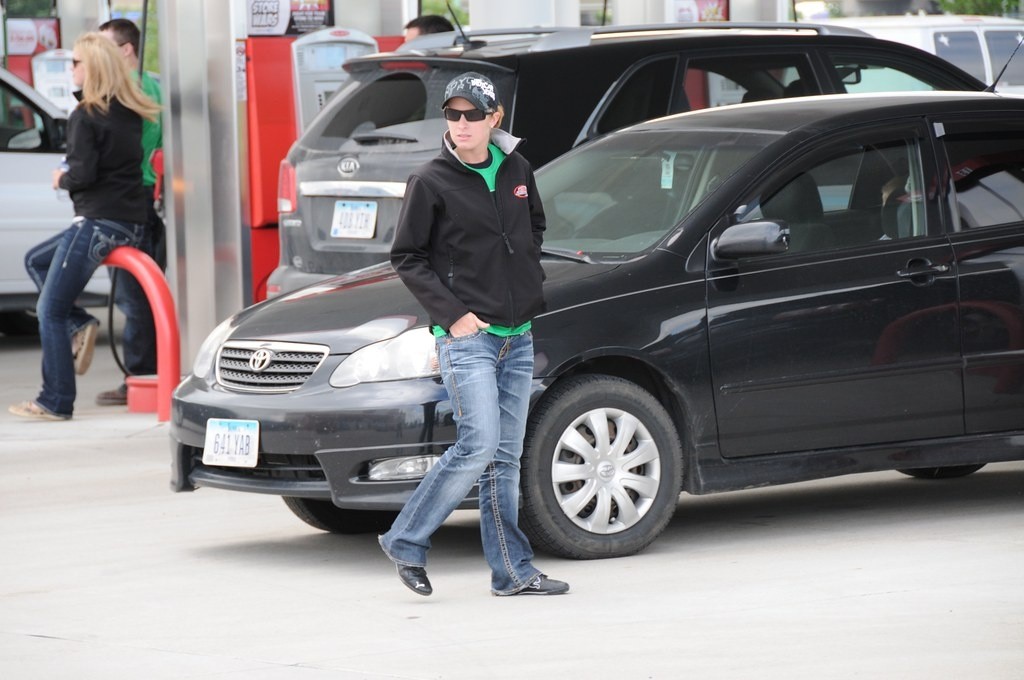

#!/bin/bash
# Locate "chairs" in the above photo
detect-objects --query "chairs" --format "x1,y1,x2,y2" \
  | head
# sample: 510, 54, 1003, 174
730, 170, 847, 256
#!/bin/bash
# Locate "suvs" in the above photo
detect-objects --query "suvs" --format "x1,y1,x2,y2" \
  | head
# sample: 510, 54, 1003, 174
265, 15, 1024, 303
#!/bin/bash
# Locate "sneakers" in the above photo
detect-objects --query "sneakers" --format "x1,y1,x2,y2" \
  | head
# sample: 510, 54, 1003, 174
516, 574, 569, 595
396, 563, 432, 596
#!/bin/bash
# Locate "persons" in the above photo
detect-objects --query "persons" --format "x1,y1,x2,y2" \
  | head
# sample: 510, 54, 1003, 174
97, 20, 168, 408
403, 14, 449, 44
378, 72, 572, 598
9, 33, 160, 421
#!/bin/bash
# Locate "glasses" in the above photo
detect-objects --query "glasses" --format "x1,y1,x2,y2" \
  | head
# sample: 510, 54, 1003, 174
73, 59, 82, 68
444, 107, 493, 121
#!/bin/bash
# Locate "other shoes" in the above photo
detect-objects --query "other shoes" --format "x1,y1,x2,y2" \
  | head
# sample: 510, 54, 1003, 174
96, 390, 127, 405
74, 323, 97, 374
10, 401, 66, 420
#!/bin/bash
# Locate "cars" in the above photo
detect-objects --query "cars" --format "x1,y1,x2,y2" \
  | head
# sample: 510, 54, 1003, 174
168, 38, 1024, 560
0, 67, 129, 315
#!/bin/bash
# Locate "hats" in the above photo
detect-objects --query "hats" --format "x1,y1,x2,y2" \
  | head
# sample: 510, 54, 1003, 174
442, 71, 501, 111
